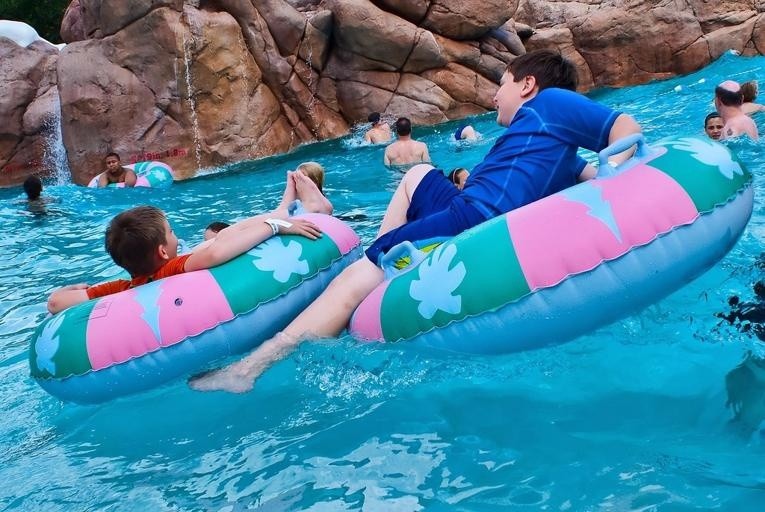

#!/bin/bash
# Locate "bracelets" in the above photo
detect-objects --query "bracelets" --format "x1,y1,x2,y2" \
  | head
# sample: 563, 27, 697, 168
265, 218, 278, 235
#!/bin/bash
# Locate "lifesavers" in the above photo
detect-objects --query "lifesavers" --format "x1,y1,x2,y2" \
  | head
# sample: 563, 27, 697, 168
29, 199, 364, 407
86, 161, 174, 188
346, 135, 754, 355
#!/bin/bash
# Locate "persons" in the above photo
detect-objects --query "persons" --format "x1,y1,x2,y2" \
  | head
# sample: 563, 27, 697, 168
96, 152, 137, 188
185, 48, 642, 393
13, 177, 47, 204
454, 124, 476, 141
447, 167, 470, 190
704, 80, 765, 142
296, 162, 326, 197
384, 117, 431, 165
204, 221, 230, 241
364, 112, 391, 144
47, 170, 333, 317
